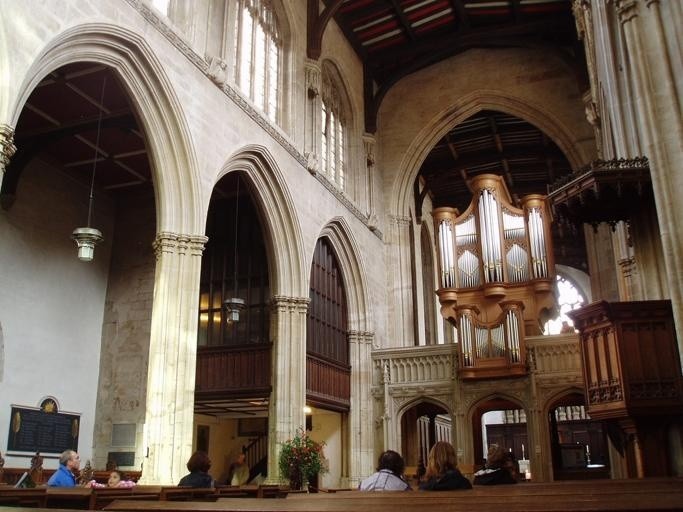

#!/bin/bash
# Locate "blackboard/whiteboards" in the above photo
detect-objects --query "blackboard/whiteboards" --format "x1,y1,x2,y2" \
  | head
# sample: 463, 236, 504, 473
5, 404, 82, 458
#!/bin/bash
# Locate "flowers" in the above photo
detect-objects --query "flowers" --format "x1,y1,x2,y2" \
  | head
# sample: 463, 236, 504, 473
274, 422, 330, 493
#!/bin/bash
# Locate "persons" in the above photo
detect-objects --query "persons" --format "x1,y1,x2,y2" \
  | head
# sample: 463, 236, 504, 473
560, 321, 574, 334
91, 469, 136, 488
47, 448, 97, 488
418, 440, 473, 491
358, 450, 413, 492
177, 450, 212, 498
473, 443, 514, 485
228, 452, 250, 486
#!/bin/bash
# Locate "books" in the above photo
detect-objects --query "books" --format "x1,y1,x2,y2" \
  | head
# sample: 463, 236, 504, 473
14, 471, 39, 488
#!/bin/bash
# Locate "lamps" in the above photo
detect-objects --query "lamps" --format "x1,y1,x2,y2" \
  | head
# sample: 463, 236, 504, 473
68, 63, 107, 262
219, 174, 245, 325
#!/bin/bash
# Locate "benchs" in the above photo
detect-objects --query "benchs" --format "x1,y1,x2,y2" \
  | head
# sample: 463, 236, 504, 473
46, 479, 683, 512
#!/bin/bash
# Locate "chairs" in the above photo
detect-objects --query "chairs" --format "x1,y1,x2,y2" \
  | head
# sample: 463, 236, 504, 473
0, 486, 48, 509
